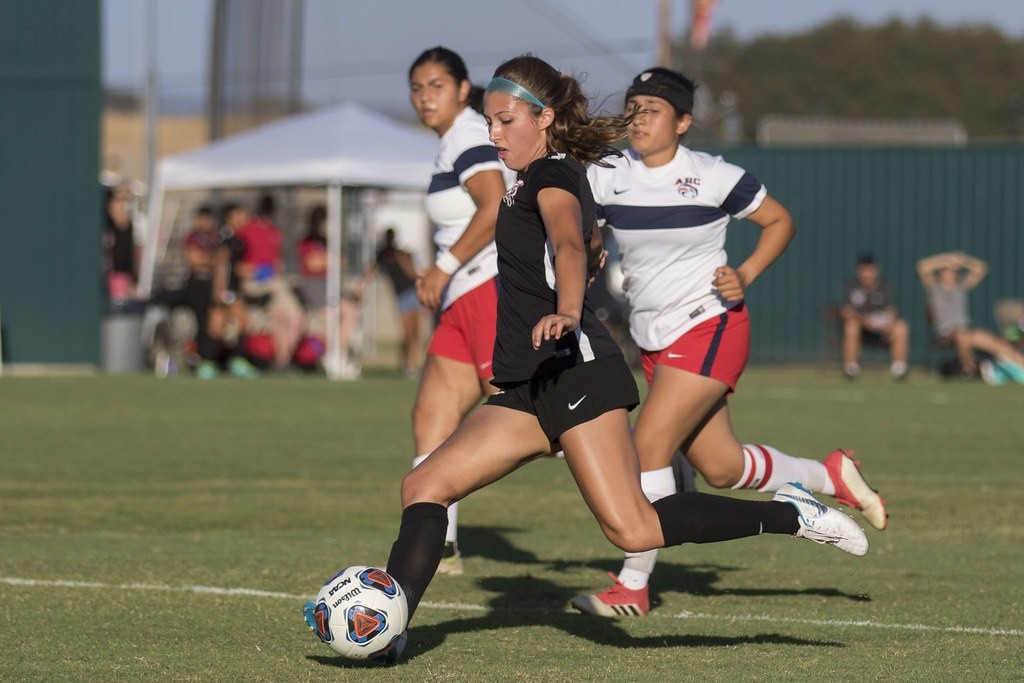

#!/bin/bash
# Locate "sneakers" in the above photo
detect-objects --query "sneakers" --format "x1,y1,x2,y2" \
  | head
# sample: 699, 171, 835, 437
771, 483, 870, 557
435, 541, 465, 576
571, 570, 651, 618
302, 601, 407, 664
823, 448, 889, 530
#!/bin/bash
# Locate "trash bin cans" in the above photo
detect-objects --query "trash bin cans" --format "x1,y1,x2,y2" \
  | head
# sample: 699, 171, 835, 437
99, 295, 148, 373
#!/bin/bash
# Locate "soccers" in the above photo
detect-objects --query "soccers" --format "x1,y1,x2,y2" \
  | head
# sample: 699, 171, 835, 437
308, 561, 414, 666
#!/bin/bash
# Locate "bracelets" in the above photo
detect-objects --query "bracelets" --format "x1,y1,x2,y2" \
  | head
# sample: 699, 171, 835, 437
435, 250, 461, 276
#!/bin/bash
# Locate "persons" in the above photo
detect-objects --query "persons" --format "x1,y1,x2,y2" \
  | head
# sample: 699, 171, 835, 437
914, 251, 1024, 381
408, 47, 698, 579
303, 51, 869, 667
571, 60, 888, 618
98, 186, 420, 379
838, 255, 909, 380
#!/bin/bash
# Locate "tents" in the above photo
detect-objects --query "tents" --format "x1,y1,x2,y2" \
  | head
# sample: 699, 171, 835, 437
139, 97, 445, 380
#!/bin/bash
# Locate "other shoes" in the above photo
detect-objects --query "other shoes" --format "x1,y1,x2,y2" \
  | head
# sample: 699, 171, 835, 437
196, 359, 218, 380
230, 356, 257, 377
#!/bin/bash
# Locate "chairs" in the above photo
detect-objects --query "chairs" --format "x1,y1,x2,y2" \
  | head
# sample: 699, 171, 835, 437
926, 311, 962, 373
832, 307, 895, 357
